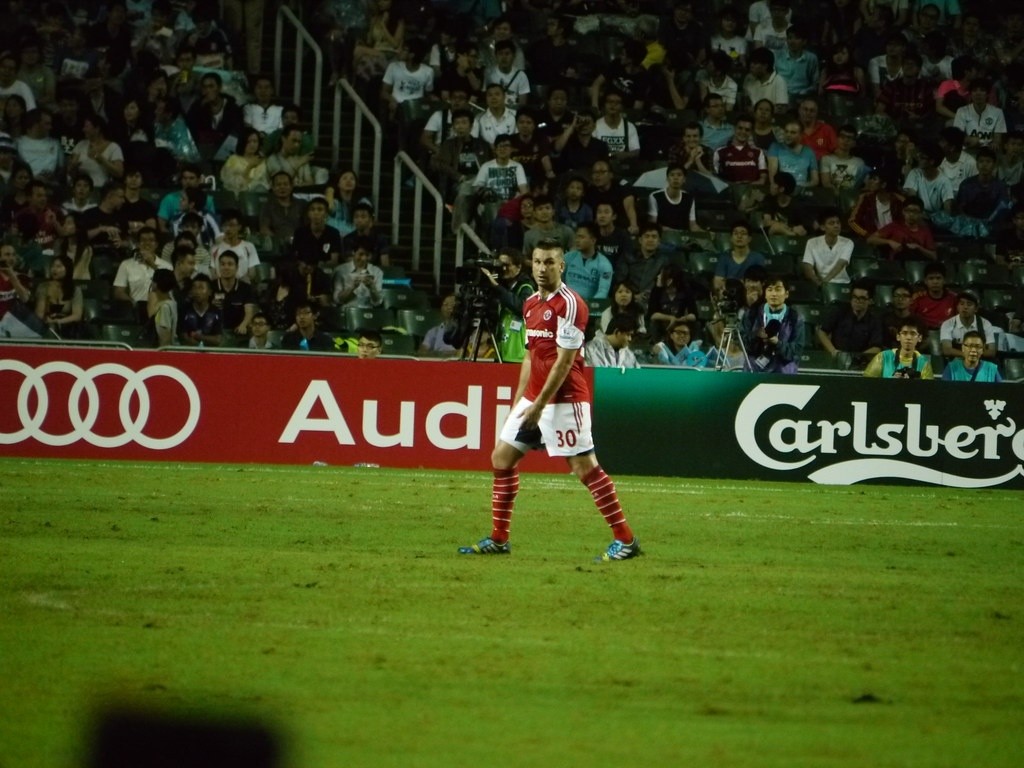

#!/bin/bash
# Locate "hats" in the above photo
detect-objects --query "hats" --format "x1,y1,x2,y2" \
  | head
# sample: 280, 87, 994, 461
957, 289, 980, 306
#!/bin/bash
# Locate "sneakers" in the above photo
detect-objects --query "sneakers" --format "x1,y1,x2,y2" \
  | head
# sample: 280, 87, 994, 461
457, 537, 511, 554
592, 536, 639, 562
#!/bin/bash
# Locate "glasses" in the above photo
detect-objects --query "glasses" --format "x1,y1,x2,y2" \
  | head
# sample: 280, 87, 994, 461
851, 295, 869, 303
252, 321, 269, 326
673, 330, 689, 335
295, 312, 313, 316
358, 344, 379, 350
591, 170, 609, 175
963, 344, 984, 350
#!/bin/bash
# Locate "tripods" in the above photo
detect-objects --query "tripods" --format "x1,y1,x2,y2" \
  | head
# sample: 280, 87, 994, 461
715, 325, 754, 372
458, 310, 502, 363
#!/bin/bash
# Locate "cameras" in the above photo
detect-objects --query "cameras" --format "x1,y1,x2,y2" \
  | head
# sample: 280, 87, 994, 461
896, 366, 921, 379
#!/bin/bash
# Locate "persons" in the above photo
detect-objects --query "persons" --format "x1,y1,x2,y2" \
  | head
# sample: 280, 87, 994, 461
482, 247, 537, 363
862, 318, 933, 380
456, 237, 642, 563
649, 319, 692, 369
801, 212, 855, 289
355, 329, 382, 359
280, 301, 335, 352
599, 280, 646, 343
176, 272, 223, 346
337, 0, 1024, 368
942, 331, 1001, 382
815, 277, 890, 366
584, 313, 641, 367
142, 268, 178, 347
0, 0, 393, 345
561, 221, 613, 315
743, 275, 806, 376
418, 294, 458, 358
940, 288, 999, 366
240, 312, 278, 350
